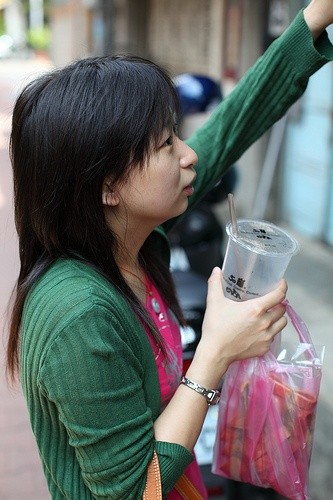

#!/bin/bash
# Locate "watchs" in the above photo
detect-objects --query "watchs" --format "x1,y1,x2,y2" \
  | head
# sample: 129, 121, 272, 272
180, 376, 220, 405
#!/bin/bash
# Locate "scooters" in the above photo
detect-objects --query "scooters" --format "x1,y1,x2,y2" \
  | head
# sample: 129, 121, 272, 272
167, 73, 225, 369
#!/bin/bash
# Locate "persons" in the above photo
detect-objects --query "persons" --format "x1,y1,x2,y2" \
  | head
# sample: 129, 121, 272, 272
156, 73, 288, 500
7, 0, 333, 500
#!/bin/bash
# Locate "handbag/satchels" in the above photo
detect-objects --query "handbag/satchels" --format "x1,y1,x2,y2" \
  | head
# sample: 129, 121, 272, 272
210, 300, 324, 499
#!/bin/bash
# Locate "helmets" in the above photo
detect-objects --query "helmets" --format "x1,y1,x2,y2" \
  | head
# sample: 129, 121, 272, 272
172, 72, 223, 119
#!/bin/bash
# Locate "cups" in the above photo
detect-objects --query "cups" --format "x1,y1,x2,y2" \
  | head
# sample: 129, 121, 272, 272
220, 218, 299, 302
